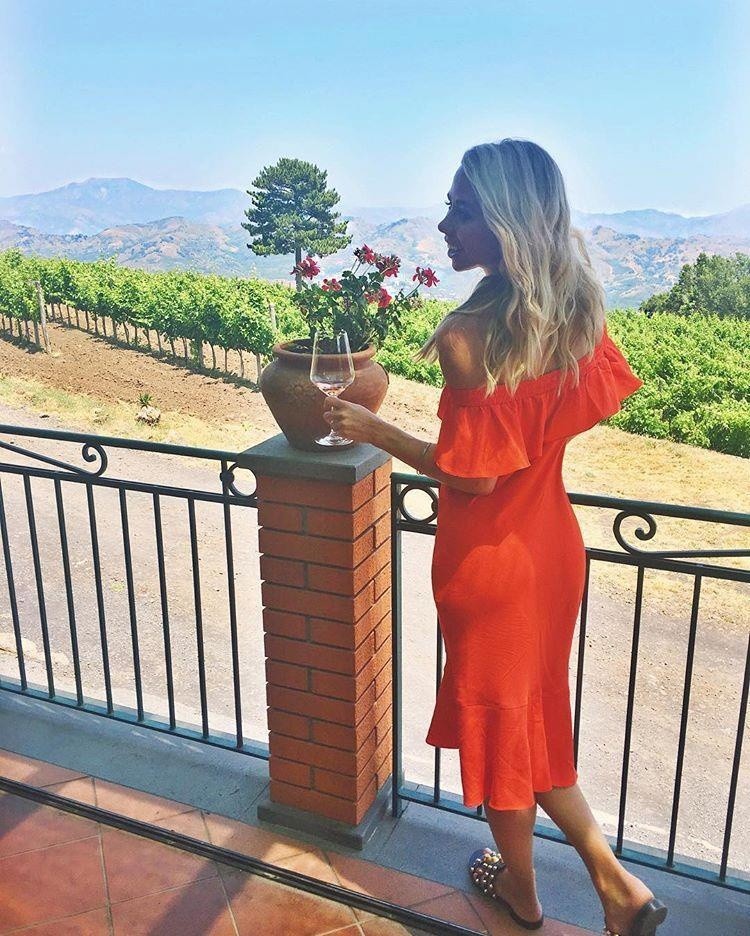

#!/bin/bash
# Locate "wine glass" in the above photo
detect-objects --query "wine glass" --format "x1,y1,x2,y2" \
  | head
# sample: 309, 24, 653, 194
309, 329, 356, 447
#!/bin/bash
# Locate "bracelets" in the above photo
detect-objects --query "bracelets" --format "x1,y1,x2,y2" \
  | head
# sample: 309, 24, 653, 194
416, 442, 432, 474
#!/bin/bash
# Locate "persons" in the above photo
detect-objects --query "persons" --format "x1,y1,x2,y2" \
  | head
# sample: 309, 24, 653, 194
322, 137, 668, 935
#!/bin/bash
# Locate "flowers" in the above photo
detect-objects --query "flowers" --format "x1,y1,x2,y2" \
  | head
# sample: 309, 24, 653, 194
288, 245, 443, 352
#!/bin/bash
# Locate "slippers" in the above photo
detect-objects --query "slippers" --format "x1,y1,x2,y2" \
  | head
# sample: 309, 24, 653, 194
608, 896, 669, 936
469, 848, 545, 930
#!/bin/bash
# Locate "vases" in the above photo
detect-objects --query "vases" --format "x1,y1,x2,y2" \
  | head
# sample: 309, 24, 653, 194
259, 336, 388, 451
